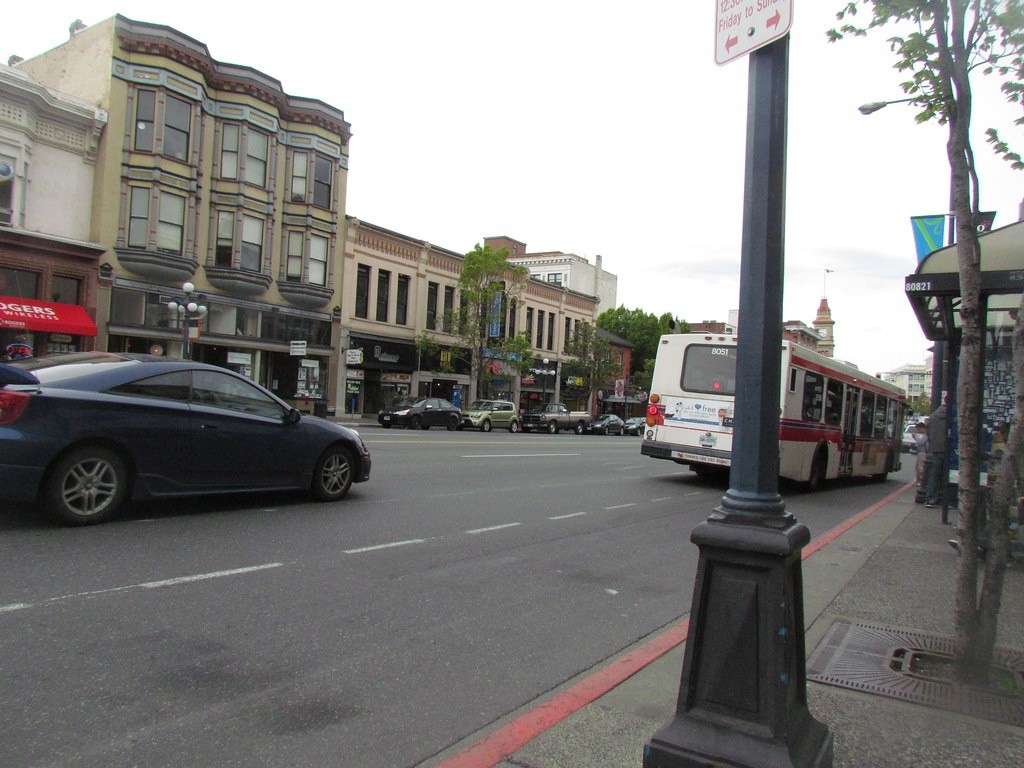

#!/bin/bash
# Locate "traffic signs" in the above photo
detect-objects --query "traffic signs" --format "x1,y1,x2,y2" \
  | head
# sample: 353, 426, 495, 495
713, 1, 795, 66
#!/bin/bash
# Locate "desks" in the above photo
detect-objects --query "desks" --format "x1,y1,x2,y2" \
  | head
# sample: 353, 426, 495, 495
283, 397, 326, 416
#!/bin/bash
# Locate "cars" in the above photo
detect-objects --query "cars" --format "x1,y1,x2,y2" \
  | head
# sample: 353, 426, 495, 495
460, 400, 519, 433
0, 352, 372, 525
377, 396, 462, 430
901, 424, 923, 453
624, 417, 647, 436
586, 414, 625, 436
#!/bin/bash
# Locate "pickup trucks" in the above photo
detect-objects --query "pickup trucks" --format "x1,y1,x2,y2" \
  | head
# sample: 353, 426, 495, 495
521, 403, 592, 435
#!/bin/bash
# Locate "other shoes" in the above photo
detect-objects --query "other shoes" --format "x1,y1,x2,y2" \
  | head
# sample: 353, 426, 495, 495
925, 500, 933, 506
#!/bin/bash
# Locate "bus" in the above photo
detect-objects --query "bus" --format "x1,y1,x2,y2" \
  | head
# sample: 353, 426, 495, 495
640, 334, 914, 493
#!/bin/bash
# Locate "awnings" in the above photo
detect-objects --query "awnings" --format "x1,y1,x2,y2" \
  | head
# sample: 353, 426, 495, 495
905, 223, 1024, 341
602, 395, 641, 403
0, 296, 97, 336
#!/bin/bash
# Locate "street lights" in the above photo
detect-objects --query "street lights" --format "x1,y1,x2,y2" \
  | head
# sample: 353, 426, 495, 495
535, 358, 556, 413
168, 282, 206, 359
858, 95, 954, 505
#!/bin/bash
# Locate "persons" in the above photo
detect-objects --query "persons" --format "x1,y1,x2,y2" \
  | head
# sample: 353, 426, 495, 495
435, 386, 440, 398
398, 390, 403, 396
912, 422, 928, 486
542, 406, 546, 411
613, 403, 625, 420
925, 396, 955, 507
442, 392, 446, 398
861, 408, 871, 431
